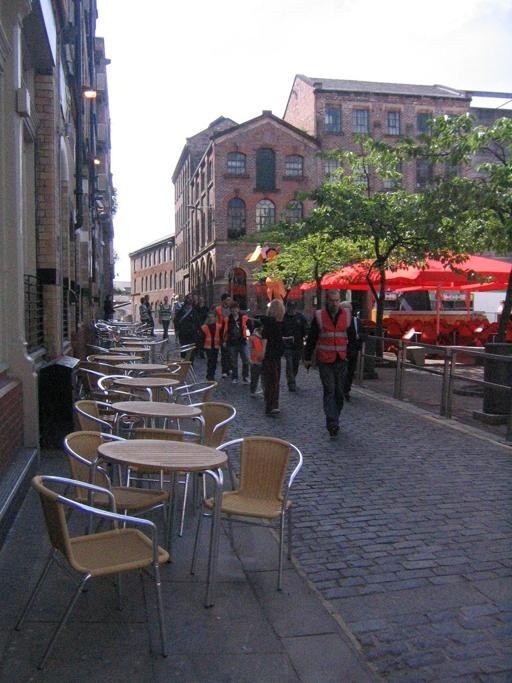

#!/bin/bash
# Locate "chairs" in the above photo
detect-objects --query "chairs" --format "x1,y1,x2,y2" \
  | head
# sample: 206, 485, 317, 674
63, 430, 170, 563
375, 315, 512, 358
191, 436, 304, 591
15, 474, 169, 670
174, 380, 218, 431
129, 427, 201, 537
168, 402, 238, 502
74, 399, 144, 489
78, 320, 196, 403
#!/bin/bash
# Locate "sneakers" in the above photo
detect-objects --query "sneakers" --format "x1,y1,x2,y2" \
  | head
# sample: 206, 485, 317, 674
265, 404, 281, 415
329, 425, 340, 437
231, 377, 240, 384
241, 376, 251, 385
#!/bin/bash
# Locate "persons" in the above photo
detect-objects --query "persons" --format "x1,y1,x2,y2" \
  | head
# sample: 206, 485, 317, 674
103, 295, 115, 320
139, 298, 151, 329
144, 295, 156, 337
262, 248, 287, 277
170, 290, 362, 439
158, 296, 173, 339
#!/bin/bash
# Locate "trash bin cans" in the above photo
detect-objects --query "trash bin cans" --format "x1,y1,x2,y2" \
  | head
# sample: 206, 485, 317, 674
37, 357, 81, 450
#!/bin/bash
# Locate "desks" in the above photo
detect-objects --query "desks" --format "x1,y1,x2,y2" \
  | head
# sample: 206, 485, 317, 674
98, 438, 229, 607
94, 322, 180, 401
110, 401, 207, 515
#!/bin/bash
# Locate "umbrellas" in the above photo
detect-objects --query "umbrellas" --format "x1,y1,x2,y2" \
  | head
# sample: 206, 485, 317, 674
299, 251, 512, 335
396, 283, 508, 320
